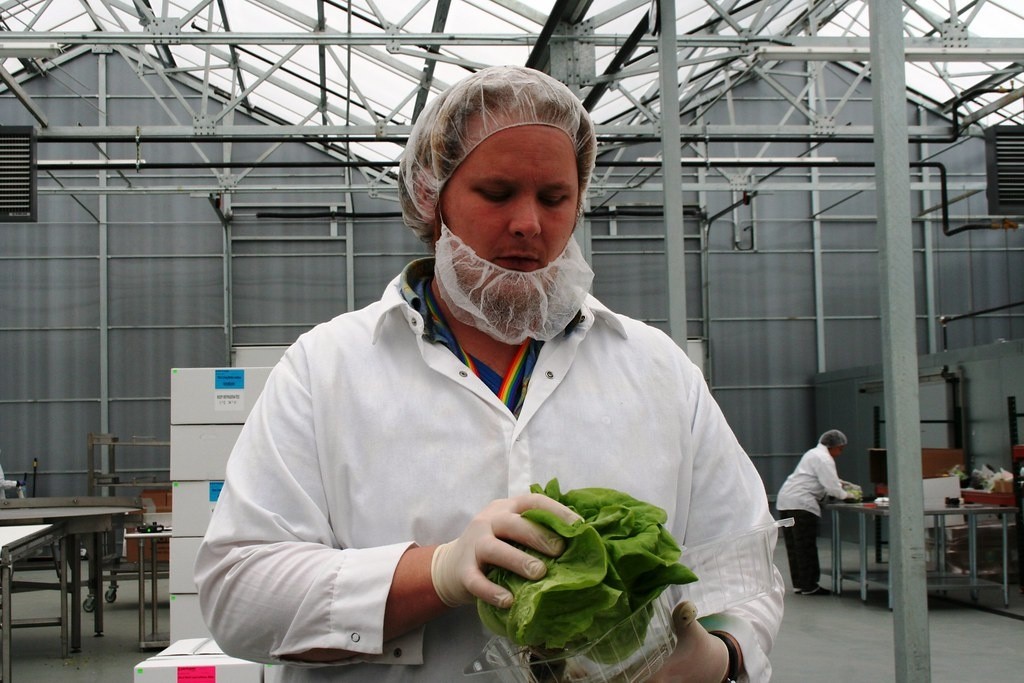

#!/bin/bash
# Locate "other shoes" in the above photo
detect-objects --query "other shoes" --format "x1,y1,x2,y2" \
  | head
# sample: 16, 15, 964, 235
792, 586, 829, 595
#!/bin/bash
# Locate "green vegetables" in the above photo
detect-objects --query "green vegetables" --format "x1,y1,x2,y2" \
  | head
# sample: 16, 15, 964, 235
476, 478, 699, 665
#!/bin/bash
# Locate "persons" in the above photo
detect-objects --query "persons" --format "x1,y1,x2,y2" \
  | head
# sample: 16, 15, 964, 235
776, 430, 856, 597
193, 65, 786, 683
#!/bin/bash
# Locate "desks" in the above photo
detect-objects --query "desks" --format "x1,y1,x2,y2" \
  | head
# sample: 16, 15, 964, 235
1, 506, 146, 653
0, 524, 67, 683
124, 527, 170, 648
820, 498, 1018, 611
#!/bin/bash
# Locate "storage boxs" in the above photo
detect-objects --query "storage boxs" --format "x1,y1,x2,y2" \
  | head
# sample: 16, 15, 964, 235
923, 477, 966, 531
133, 344, 283, 683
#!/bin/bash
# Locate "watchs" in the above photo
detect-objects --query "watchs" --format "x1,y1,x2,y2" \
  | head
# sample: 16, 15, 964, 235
708, 631, 739, 683
